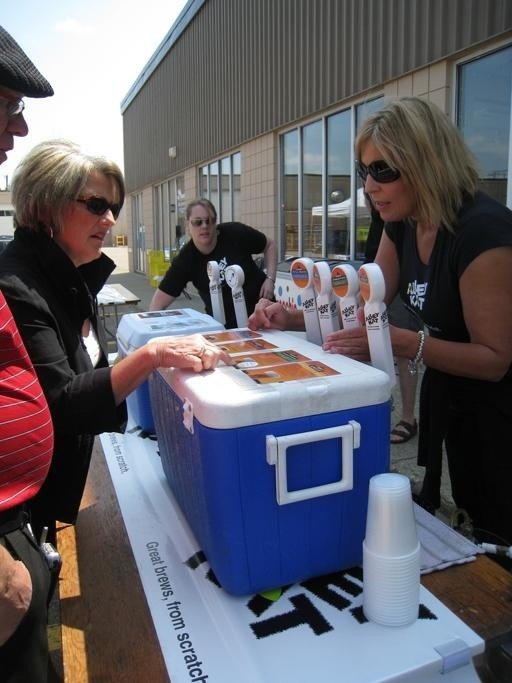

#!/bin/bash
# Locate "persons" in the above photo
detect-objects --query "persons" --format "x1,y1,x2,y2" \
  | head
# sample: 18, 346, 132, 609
148, 198, 278, 330
248, 96, 511, 572
0, 136, 237, 608
362, 183, 426, 446
0, 23, 65, 683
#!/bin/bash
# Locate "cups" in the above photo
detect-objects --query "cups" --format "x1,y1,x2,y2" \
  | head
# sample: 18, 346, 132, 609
361, 472, 422, 629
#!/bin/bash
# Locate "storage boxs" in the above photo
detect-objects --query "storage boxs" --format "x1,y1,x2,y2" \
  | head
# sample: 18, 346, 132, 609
117, 307, 394, 598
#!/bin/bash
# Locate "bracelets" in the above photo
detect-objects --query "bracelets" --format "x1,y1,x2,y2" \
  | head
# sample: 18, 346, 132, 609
265, 276, 275, 283
406, 330, 426, 377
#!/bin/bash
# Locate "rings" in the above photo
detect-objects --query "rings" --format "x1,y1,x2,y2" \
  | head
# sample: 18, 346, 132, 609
198, 347, 205, 357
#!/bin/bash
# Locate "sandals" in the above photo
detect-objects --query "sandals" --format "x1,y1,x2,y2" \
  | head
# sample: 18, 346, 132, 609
390, 420, 417, 444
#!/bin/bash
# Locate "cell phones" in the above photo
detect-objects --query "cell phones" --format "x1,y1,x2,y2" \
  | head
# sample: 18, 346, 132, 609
39, 526, 62, 606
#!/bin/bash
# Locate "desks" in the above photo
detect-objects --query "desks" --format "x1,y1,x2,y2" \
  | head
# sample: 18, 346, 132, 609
58, 351, 512, 682
94, 282, 142, 329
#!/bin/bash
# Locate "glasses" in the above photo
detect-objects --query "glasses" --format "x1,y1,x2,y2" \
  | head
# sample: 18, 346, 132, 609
351, 159, 402, 184
75, 194, 121, 217
187, 217, 216, 229
1, 97, 28, 112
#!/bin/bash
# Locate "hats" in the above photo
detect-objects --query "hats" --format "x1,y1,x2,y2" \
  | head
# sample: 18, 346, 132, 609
0, 25, 55, 98
